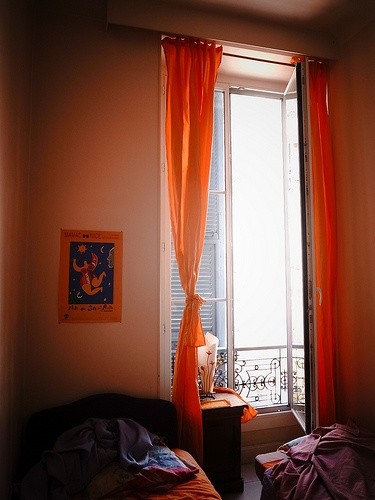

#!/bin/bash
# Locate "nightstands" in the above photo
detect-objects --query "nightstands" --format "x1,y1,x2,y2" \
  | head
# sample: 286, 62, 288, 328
202, 393, 245, 494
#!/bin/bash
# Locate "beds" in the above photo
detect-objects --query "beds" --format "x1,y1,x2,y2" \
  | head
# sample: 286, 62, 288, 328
6, 392, 222, 500
254, 425, 375, 500
